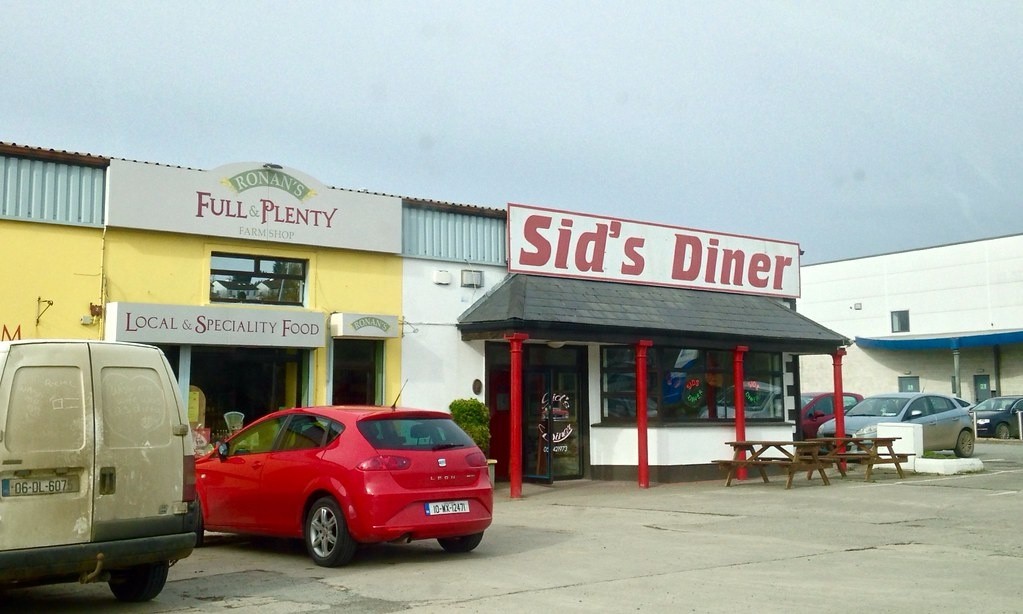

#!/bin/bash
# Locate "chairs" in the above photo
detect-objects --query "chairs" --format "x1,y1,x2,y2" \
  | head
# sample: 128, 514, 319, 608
885, 401, 897, 413
410, 422, 436, 445
1005, 405, 1011, 410
294, 426, 331, 449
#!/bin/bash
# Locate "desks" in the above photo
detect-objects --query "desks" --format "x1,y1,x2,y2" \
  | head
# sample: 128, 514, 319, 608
723, 441, 831, 490
803, 437, 905, 482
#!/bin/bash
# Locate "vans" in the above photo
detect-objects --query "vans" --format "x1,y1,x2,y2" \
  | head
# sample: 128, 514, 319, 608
0, 338, 197, 605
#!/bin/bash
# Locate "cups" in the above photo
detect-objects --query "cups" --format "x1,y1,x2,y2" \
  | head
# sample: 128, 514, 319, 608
223, 411, 245, 437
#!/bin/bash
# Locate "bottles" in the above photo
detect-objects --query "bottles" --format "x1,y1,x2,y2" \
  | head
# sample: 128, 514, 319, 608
211, 429, 228, 442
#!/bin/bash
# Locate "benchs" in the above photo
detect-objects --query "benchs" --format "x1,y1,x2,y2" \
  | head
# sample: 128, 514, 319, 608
711, 453, 917, 471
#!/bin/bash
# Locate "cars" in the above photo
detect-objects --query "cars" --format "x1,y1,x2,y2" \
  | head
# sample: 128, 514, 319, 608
968, 394, 1023, 440
534, 346, 784, 423
195, 405, 493, 569
801, 392, 866, 456
816, 392, 976, 459
888, 394, 976, 413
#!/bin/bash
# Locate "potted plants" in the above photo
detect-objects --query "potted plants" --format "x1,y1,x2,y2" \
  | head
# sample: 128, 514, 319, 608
450, 398, 499, 487
